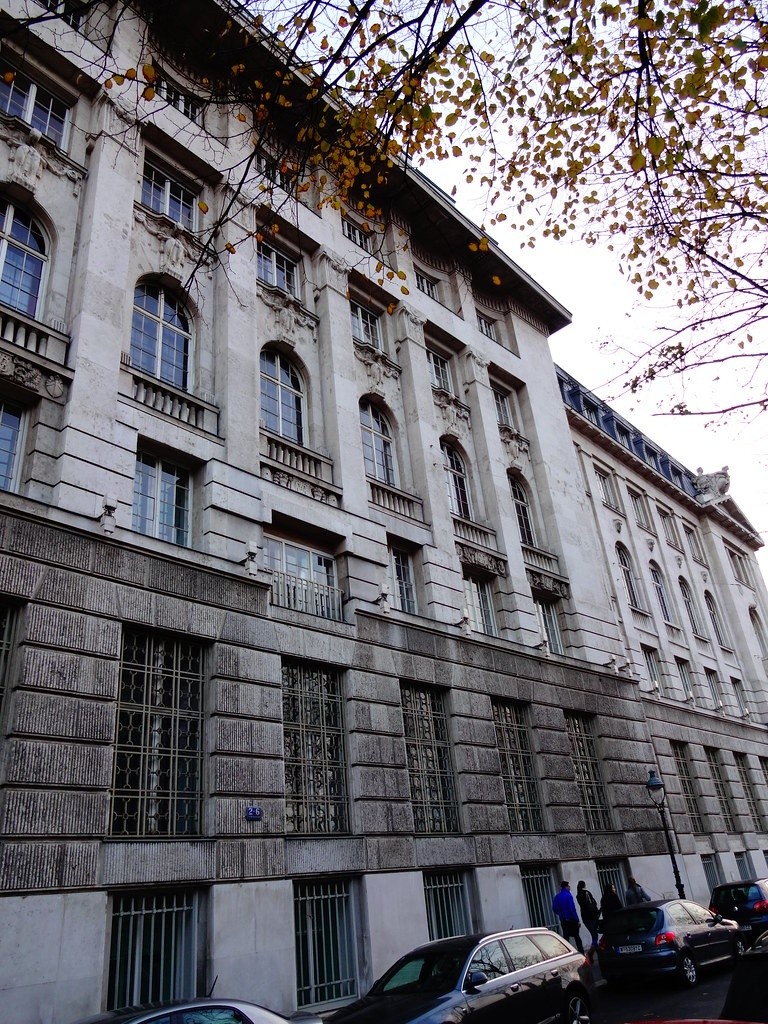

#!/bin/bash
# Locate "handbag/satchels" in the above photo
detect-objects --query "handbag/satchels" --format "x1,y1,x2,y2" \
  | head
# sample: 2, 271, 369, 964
596, 920, 604, 934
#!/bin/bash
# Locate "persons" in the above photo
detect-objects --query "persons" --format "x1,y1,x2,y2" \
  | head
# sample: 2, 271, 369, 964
602, 884, 624, 916
576, 880, 600, 965
552, 879, 585, 956
625, 878, 652, 906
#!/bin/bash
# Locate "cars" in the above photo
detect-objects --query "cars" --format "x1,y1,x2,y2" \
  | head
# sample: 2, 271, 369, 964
67, 999, 325, 1024
707, 878, 768, 955
595, 899, 746, 988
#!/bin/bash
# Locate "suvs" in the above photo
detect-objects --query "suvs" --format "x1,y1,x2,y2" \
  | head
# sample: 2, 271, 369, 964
319, 926, 611, 1024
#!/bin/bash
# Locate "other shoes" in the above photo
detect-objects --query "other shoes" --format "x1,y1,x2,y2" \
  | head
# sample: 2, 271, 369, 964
588, 952, 594, 963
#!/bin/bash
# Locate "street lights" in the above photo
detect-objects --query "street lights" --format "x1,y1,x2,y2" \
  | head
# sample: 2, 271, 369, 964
645, 769, 685, 900
